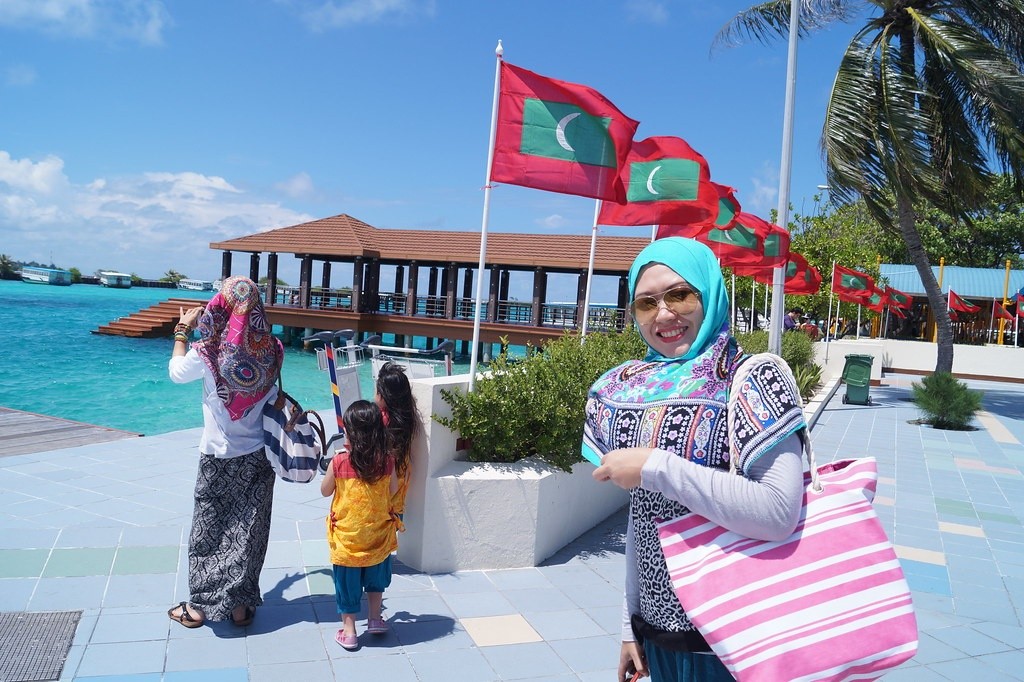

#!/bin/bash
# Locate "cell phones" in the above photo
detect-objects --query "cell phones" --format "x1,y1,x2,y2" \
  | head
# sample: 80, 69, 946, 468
623, 657, 647, 681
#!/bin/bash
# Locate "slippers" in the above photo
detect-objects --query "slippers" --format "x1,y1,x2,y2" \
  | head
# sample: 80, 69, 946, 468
168, 601, 202, 627
230, 604, 257, 624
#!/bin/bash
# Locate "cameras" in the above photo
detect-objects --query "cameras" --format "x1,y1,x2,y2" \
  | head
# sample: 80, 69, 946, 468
196, 308, 205, 325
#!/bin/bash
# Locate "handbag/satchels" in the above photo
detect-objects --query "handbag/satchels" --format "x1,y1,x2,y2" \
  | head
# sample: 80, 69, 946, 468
263, 335, 327, 483
656, 353, 920, 682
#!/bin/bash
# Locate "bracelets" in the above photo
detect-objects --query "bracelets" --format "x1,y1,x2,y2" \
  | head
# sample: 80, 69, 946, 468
174, 336, 188, 342
172, 332, 189, 339
174, 323, 192, 335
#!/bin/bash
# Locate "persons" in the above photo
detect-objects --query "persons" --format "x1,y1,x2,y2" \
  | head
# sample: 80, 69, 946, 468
579, 235, 810, 682
801, 313, 819, 343
167, 276, 284, 629
782, 306, 802, 333
320, 399, 402, 649
340, 361, 421, 532
820, 312, 848, 339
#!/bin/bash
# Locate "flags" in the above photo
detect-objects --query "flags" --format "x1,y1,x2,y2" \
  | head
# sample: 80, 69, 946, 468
886, 286, 913, 309
859, 286, 890, 314
1016, 294, 1024, 316
948, 305, 959, 320
890, 303, 908, 320
832, 264, 875, 298
693, 211, 773, 263
949, 290, 981, 313
719, 223, 791, 269
651, 182, 740, 239
491, 59, 641, 207
994, 301, 1014, 320
594, 135, 717, 227
754, 251, 807, 290
840, 292, 876, 306
781, 266, 822, 297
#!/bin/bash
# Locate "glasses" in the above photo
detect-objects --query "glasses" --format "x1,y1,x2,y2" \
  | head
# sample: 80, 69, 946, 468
628, 285, 700, 325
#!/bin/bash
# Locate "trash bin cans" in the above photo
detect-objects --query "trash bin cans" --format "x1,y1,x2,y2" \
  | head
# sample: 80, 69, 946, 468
840, 353, 875, 406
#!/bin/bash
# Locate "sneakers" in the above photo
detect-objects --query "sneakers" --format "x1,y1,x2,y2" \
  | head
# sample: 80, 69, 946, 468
367, 617, 388, 633
334, 629, 358, 649
